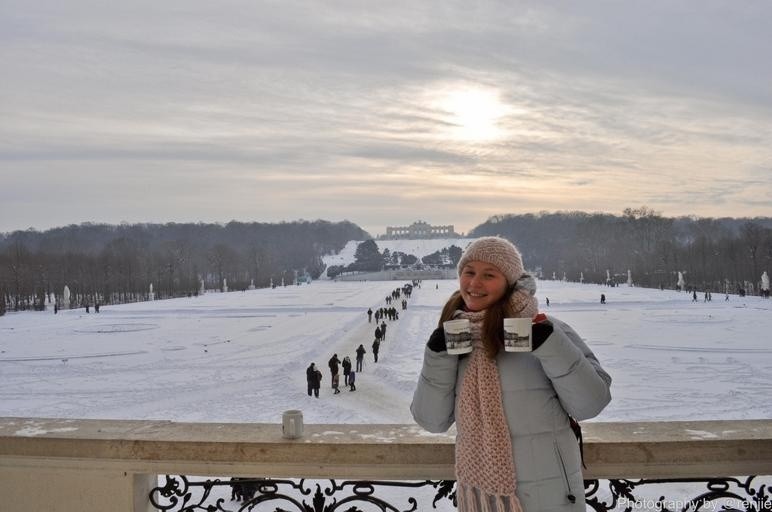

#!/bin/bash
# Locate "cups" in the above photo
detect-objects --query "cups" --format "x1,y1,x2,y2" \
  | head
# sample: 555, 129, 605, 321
502, 318, 534, 352
282, 410, 305, 439
441, 319, 474, 355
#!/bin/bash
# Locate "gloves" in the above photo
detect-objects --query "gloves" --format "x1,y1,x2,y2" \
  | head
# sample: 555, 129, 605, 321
532, 320, 553, 351
429, 328, 468, 360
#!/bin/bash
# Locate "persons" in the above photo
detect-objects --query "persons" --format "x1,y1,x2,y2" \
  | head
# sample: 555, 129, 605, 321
86, 303, 89, 312
660, 284, 769, 303
410, 237, 614, 512
342, 356, 352, 386
244, 287, 245, 292
368, 279, 423, 324
329, 353, 341, 388
306, 362, 316, 396
381, 321, 387, 340
372, 339, 379, 362
436, 283, 440, 293
94, 304, 100, 313
375, 327, 381, 345
271, 279, 312, 290
333, 371, 340, 394
242, 287, 243, 293
54, 304, 57, 315
349, 368, 356, 391
545, 296, 549, 307
355, 344, 367, 372
312, 367, 322, 397
601, 294, 606, 304
184, 288, 235, 299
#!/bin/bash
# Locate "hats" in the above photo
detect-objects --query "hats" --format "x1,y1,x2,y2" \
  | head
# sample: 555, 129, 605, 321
458, 235, 523, 285
